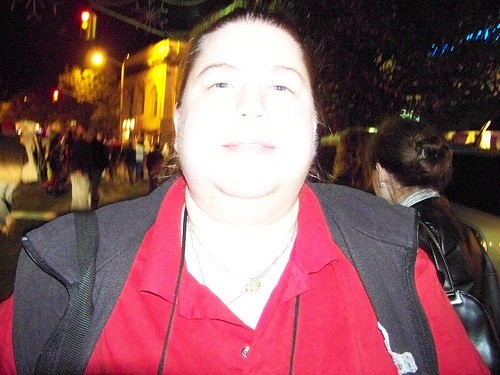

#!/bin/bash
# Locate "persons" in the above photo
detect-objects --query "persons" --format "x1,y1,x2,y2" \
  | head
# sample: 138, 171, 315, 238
330, 118, 500, 375
0, 5, 491, 375
32, 122, 164, 209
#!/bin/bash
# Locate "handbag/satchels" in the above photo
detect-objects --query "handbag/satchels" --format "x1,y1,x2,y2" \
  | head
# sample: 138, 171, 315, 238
419, 221, 500, 369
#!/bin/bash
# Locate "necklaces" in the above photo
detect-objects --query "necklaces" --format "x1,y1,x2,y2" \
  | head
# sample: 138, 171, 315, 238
187, 218, 297, 307
